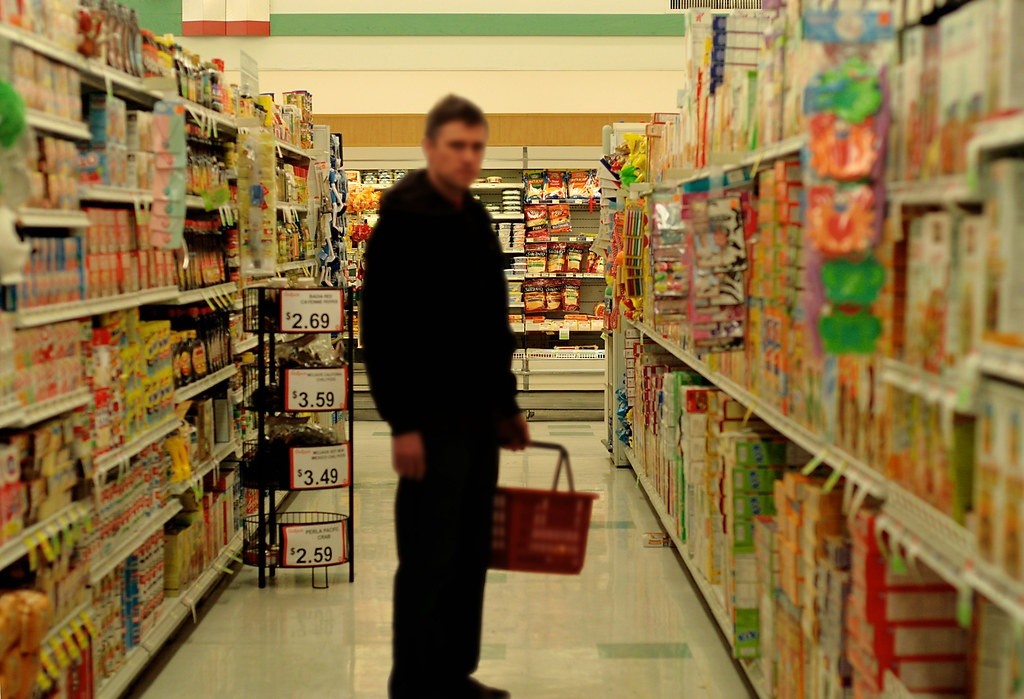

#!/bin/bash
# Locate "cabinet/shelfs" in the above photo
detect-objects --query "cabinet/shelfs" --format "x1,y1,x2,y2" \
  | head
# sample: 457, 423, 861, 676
0, 0, 348, 699
344, 146, 607, 391
589, 0, 1024, 699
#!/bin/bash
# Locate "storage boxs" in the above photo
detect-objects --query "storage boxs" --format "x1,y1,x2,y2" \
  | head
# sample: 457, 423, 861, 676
0, 39, 180, 699
623, 0, 1024, 699
160, 463, 235, 591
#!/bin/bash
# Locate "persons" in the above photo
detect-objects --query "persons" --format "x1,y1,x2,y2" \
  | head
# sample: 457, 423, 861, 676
356, 94, 530, 698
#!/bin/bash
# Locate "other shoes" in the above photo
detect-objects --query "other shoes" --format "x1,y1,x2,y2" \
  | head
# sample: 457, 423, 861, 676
450, 678, 510, 699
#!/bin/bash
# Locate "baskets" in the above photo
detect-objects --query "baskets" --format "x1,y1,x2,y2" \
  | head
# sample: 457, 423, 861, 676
487, 439, 597, 576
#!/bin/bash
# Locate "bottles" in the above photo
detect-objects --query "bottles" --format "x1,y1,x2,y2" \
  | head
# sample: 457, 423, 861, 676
79, 1, 231, 447
